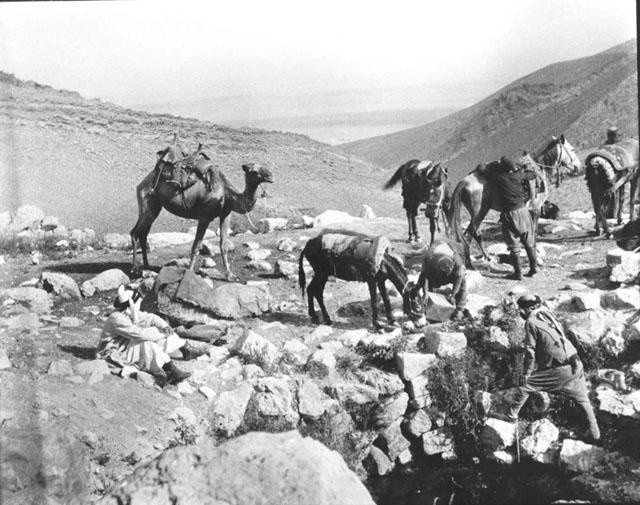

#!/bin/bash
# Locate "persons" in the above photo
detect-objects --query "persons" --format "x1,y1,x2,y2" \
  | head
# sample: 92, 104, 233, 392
602, 125, 622, 220
489, 293, 606, 447
474, 155, 539, 281
417, 238, 470, 323
94, 286, 210, 385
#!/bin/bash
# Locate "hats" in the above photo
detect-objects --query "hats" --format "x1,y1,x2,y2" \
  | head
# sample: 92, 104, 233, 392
501, 155, 515, 170
436, 256, 455, 277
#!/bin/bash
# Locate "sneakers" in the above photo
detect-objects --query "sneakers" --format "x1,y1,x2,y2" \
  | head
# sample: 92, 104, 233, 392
449, 310, 465, 322
491, 410, 518, 424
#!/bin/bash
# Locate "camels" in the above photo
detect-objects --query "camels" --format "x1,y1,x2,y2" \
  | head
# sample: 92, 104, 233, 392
130, 154, 273, 282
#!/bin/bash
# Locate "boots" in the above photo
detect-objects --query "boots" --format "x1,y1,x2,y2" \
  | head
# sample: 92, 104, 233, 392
523, 245, 538, 278
505, 252, 523, 280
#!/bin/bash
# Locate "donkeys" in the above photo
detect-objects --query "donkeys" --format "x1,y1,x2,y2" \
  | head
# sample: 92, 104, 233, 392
298, 228, 427, 334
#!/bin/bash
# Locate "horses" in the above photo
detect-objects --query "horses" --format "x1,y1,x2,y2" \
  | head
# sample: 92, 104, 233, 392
584, 135, 640, 240
447, 133, 581, 270
384, 159, 462, 243
